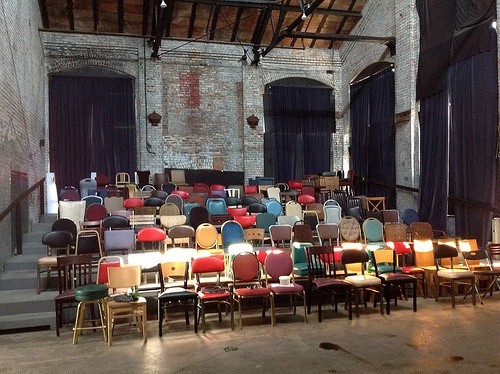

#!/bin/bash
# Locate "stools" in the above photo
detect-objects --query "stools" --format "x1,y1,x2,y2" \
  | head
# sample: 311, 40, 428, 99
71, 284, 109, 345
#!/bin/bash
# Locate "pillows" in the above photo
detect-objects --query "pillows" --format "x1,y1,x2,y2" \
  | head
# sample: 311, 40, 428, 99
343, 204, 363, 223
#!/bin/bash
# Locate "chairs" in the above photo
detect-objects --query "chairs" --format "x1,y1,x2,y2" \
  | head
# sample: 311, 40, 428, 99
35, 173, 500, 348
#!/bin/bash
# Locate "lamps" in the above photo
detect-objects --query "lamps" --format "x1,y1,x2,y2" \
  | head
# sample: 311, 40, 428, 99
245, 112, 260, 131
146, 110, 162, 127
160, 0, 167, 8
301, 10, 308, 21
